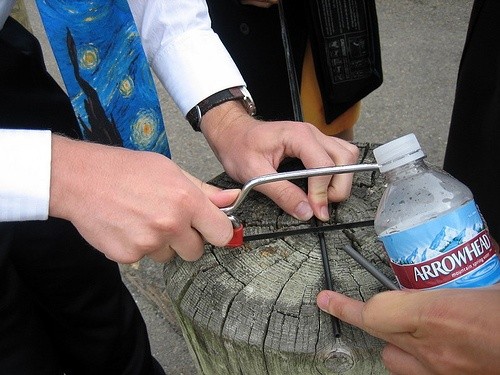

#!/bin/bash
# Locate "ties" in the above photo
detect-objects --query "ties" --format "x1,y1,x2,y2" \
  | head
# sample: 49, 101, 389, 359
35, 0, 171, 159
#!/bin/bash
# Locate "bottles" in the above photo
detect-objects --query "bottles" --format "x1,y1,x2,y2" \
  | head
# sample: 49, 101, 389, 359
372, 133, 500, 290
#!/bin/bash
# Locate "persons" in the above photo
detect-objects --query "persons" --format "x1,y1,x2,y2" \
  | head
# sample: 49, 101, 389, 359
203, 0, 384, 143
0, 0, 360, 375
317, 0, 500, 375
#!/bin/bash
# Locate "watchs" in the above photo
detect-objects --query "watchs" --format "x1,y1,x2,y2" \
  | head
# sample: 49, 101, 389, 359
186, 84, 256, 133
